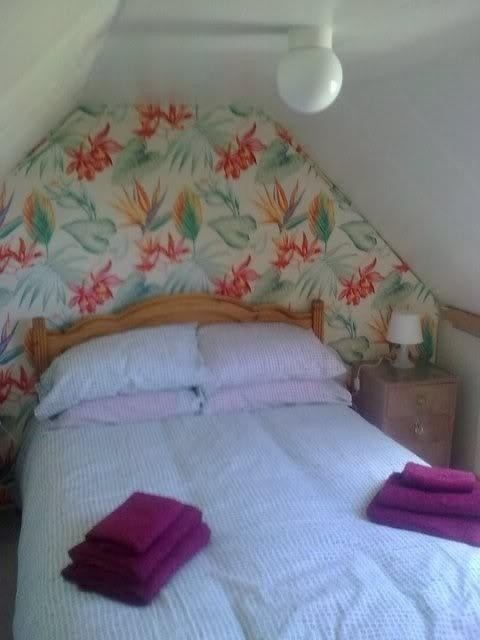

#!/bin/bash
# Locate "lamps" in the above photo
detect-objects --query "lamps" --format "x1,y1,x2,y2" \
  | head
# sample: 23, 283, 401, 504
383, 310, 424, 370
275, 23, 343, 114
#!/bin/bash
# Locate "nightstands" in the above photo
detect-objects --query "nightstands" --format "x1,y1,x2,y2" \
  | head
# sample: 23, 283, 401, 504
351, 361, 459, 479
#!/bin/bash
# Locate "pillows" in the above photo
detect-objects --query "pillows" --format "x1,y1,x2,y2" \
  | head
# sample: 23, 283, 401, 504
206, 381, 353, 417
60, 389, 200, 424
199, 323, 347, 392
35, 320, 203, 420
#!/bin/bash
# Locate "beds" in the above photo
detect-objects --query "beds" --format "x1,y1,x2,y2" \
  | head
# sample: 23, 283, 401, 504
11, 297, 478, 640
